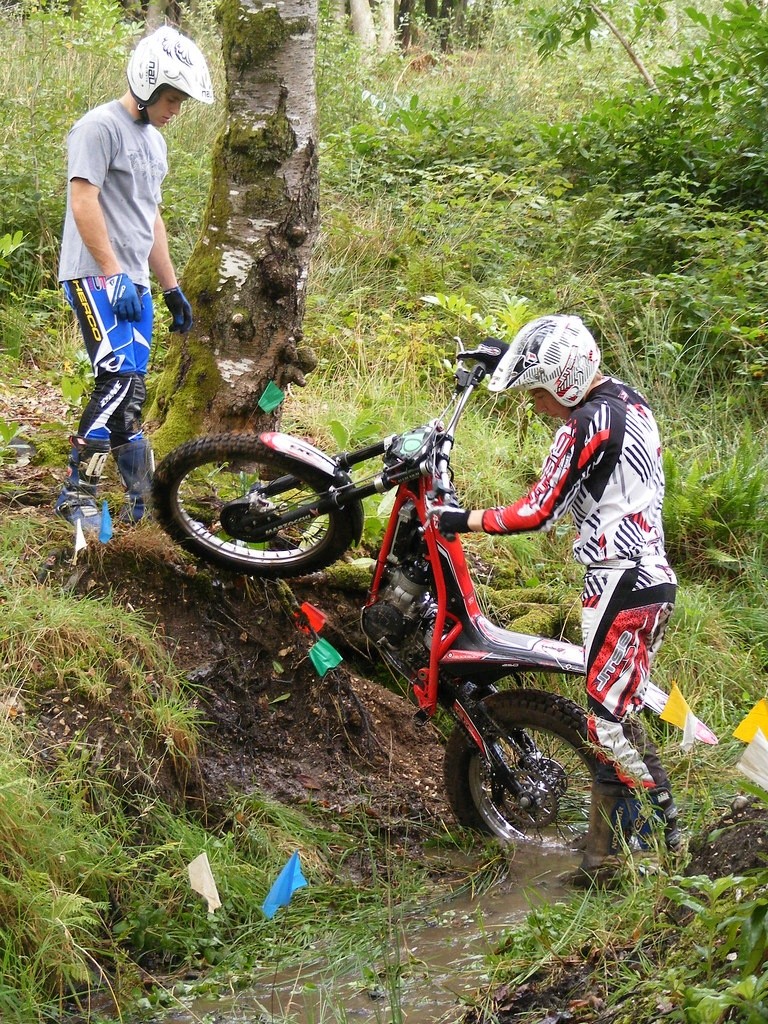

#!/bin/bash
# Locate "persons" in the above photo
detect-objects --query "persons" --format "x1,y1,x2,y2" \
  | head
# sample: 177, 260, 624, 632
425, 313, 696, 900
54, 22, 220, 554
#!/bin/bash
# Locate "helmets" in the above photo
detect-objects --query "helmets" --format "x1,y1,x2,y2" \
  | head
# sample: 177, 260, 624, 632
126, 25, 214, 107
486, 313, 602, 409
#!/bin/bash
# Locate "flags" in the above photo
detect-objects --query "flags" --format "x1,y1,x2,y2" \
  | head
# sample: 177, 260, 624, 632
97, 501, 114, 546
186, 851, 223, 921
261, 851, 311, 924
734, 728, 768, 801
293, 601, 327, 635
307, 634, 344, 678
659, 683, 696, 728
680, 709, 698, 757
257, 382, 286, 417
730, 696, 768, 746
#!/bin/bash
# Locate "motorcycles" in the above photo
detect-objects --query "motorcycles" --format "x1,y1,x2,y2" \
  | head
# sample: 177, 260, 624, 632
149, 331, 718, 850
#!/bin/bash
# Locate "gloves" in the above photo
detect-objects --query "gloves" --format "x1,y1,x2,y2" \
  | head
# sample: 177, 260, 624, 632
425, 505, 473, 538
457, 336, 510, 374
105, 272, 145, 323
163, 286, 194, 333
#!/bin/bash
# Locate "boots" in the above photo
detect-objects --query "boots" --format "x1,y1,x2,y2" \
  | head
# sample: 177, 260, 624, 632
52, 436, 112, 533
554, 784, 633, 891
634, 787, 685, 869
111, 439, 176, 523
